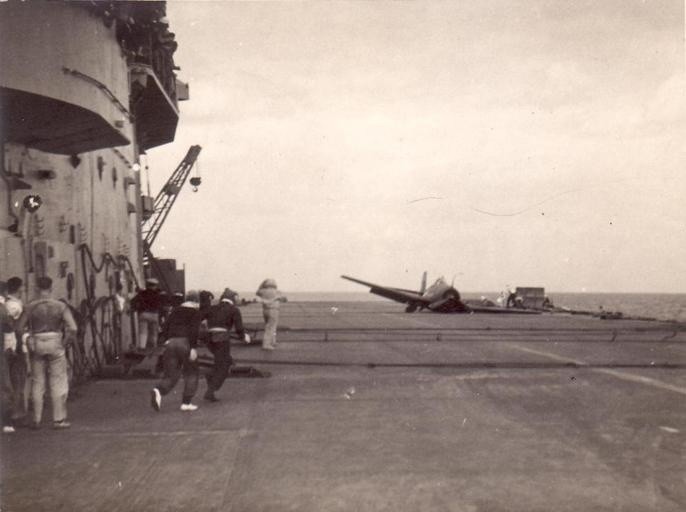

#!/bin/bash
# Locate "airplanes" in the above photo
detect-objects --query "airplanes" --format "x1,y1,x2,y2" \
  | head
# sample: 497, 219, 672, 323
341, 272, 540, 315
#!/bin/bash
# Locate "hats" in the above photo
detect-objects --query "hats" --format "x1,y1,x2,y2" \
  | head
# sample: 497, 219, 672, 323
4, 299, 23, 320
146, 278, 159, 285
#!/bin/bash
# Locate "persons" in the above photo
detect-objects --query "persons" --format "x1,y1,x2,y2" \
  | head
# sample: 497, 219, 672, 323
2, 274, 34, 426
129, 275, 168, 351
0, 278, 32, 436
505, 282, 528, 310
198, 286, 217, 324
14, 276, 79, 430
256, 278, 289, 351
592, 304, 608, 318
156, 287, 169, 333
170, 288, 185, 308
496, 291, 505, 308
203, 287, 252, 404
147, 293, 202, 412
113, 282, 126, 353
540, 293, 551, 309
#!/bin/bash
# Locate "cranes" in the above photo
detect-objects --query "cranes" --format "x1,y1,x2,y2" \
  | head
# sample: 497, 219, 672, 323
142, 145, 202, 248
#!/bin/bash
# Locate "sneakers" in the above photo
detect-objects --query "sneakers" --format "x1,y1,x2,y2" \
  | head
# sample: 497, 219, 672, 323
51, 421, 71, 429
151, 388, 162, 411
204, 392, 217, 402
180, 402, 198, 411
2, 414, 42, 434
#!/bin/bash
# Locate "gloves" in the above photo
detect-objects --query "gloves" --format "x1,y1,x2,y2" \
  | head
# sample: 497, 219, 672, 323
244, 332, 251, 343
189, 348, 197, 361
